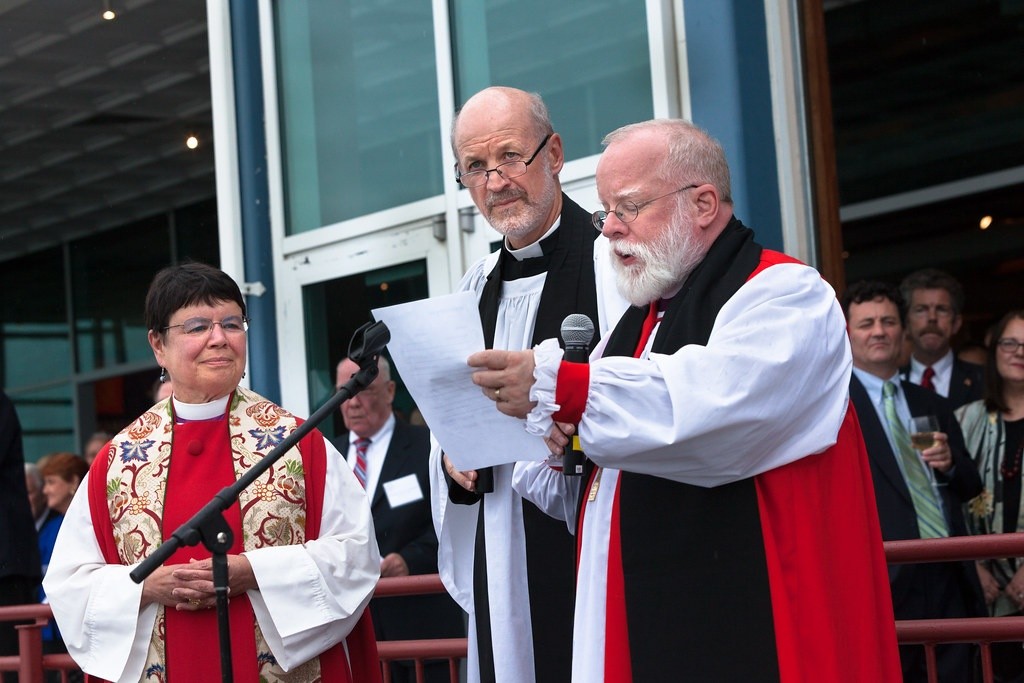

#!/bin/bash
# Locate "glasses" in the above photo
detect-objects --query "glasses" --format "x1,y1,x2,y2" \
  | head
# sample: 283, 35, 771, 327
455, 133, 550, 189
159, 316, 245, 337
914, 306, 950, 320
590, 183, 700, 232
994, 338, 1023, 353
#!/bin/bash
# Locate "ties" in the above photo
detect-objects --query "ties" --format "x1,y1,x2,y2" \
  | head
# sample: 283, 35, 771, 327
352, 437, 371, 493
919, 366, 935, 389
882, 381, 949, 538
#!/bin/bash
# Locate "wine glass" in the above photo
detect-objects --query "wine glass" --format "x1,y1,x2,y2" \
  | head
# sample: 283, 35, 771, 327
907, 415, 947, 490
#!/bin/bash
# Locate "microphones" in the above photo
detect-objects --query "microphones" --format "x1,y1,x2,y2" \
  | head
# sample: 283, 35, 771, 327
560, 314, 595, 477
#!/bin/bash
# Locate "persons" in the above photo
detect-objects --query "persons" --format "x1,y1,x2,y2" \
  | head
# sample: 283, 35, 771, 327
0, 372, 174, 683
330, 349, 465, 683
839, 268, 1024, 683
467, 118, 904, 683
43, 262, 386, 683
426, 84, 605, 683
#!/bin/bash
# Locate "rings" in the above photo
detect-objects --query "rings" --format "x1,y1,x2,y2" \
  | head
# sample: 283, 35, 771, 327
192, 599, 200, 605
494, 390, 500, 400
